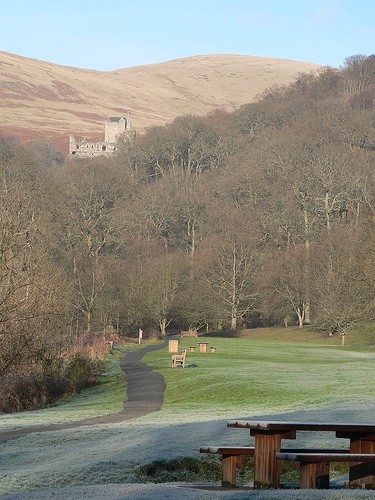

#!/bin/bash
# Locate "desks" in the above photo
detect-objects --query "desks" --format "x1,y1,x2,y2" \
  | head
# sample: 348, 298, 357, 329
227, 420, 375, 489
197, 342, 209, 353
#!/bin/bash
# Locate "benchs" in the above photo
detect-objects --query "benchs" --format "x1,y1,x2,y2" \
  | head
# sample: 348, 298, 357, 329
172, 349, 186, 369
276, 453, 375, 490
190, 346, 195, 353
210, 347, 216, 353
200, 446, 351, 489
